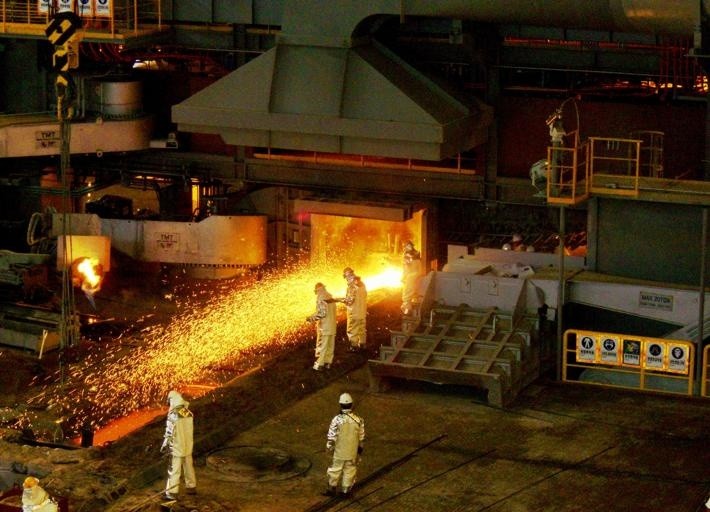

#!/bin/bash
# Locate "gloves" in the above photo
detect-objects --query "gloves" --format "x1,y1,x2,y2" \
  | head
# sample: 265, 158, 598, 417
161, 440, 168, 451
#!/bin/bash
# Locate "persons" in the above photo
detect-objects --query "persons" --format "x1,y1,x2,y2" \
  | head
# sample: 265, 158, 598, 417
21, 476, 61, 512
306, 282, 335, 372
160, 389, 197, 501
400, 240, 422, 316
501, 233, 535, 253
321, 393, 366, 497
333, 267, 368, 354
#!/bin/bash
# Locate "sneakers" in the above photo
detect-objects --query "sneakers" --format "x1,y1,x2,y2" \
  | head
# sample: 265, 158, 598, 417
358, 344, 367, 350
324, 363, 331, 369
309, 366, 323, 372
184, 487, 196, 494
346, 346, 358, 352
160, 494, 176, 500
337, 491, 347, 498
320, 490, 335, 497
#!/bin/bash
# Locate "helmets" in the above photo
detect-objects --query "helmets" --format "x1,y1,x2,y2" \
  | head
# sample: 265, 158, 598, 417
23, 475, 39, 488
401, 241, 413, 250
339, 393, 353, 404
344, 267, 354, 277
314, 283, 325, 292
